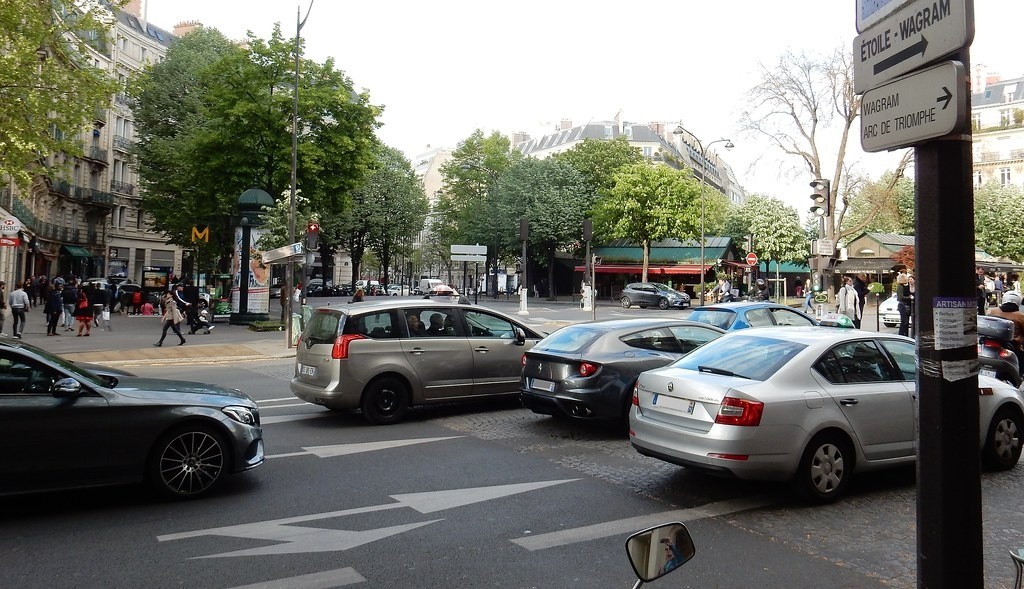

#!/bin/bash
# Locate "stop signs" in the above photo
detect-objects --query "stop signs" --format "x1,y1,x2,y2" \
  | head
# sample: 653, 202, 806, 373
747, 252, 758, 266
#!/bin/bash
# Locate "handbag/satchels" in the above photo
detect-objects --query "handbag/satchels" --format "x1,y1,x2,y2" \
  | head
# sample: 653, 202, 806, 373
173, 308, 184, 323
78, 292, 88, 308
834, 286, 847, 308
103, 307, 110, 321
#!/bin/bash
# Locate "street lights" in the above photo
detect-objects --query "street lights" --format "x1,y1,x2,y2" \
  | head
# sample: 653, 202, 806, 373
460, 164, 498, 299
672, 125, 735, 306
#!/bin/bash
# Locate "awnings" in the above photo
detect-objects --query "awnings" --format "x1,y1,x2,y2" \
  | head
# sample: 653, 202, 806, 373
575, 263, 712, 274
65, 246, 90, 256
832, 258, 900, 273
760, 261, 810, 272
727, 262, 748, 267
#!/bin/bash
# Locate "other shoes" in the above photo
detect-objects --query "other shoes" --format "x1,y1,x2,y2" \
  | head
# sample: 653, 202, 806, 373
12, 335, 19, 339
95, 319, 99, 327
0, 333, 8, 336
16, 333, 22, 338
68, 327, 74, 331
77, 333, 83, 337
153, 342, 162, 347
208, 326, 215, 330
47, 332, 54, 335
65, 329, 69, 331
178, 338, 186, 345
83, 333, 90, 336
53, 332, 61, 335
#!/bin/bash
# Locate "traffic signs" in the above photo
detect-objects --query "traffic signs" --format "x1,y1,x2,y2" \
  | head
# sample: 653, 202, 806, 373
853, 0, 976, 95
860, 60, 969, 154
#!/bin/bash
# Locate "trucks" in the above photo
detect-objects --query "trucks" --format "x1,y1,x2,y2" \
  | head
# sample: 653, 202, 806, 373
477, 272, 518, 295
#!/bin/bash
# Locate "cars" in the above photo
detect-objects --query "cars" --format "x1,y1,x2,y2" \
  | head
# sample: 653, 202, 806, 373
306, 279, 424, 297
628, 314, 1024, 508
879, 293, 912, 328
674, 301, 820, 348
269, 283, 284, 298
620, 282, 691, 310
519, 317, 731, 432
0, 334, 269, 504
74, 277, 212, 307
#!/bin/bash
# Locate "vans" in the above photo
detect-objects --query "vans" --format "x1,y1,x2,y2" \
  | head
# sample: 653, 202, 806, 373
419, 279, 443, 295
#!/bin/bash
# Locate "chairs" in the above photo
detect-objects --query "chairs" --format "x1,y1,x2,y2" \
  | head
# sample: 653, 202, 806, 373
417, 320, 428, 336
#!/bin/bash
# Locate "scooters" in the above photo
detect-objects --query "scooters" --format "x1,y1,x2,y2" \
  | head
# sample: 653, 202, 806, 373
976, 314, 1024, 389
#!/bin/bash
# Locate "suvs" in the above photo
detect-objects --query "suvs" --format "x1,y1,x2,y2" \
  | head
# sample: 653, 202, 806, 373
290, 293, 550, 426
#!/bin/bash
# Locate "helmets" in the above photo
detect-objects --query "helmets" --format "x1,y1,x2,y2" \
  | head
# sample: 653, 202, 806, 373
756, 279, 764, 286
1002, 291, 1021, 307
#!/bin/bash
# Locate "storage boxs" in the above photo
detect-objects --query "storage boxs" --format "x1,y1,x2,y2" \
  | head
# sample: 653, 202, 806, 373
977, 315, 1014, 341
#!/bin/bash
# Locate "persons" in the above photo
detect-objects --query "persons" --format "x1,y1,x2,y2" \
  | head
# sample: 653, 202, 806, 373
370, 285, 376, 296
976, 266, 1024, 375
711, 278, 733, 302
428, 314, 443, 331
794, 276, 816, 314
352, 289, 364, 302
657, 537, 684, 574
407, 315, 419, 337
534, 285, 539, 298
896, 274, 916, 337
0, 270, 215, 346
838, 272, 872, 329
280, 284, 285, 321
679, 283, 685, 292
747, 279, 769, 301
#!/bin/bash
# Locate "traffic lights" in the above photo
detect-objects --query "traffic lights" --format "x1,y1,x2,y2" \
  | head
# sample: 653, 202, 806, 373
809, 178, 830, 218
835, 247, 847, 261
306, 223, 318, 251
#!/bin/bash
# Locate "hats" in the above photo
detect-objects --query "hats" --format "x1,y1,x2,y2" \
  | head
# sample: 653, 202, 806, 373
201, 310, 208, 313
0, 281, 7, 286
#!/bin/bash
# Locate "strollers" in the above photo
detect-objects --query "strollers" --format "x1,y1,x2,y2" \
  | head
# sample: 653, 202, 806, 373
184, 303, 211, 335
